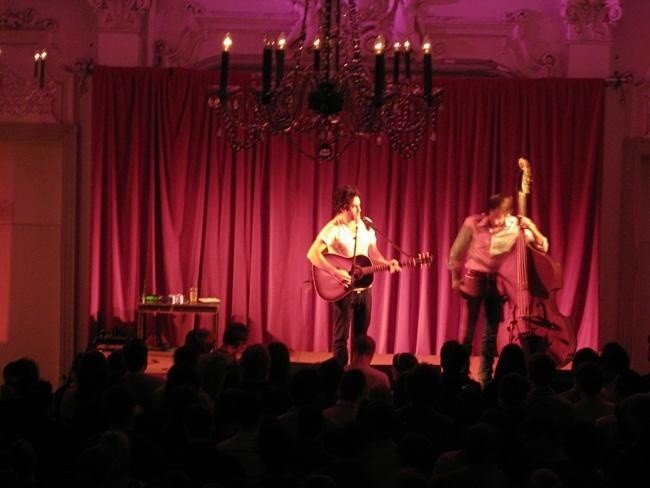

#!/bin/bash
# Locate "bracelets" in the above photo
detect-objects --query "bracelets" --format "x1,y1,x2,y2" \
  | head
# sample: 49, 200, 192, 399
449, 260, 463, 271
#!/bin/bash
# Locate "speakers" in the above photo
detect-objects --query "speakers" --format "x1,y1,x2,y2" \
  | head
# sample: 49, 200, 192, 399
371, 364, 442, 389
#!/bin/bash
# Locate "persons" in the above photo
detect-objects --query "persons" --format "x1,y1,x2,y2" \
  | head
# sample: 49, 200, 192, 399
447, 192, 549, 389
306, 187, 400, 366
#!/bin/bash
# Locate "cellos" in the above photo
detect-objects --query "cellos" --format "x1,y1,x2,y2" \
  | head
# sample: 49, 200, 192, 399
495, 157, 577, 370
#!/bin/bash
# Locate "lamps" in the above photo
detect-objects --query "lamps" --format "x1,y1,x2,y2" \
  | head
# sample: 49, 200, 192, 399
208, 0, 444, 161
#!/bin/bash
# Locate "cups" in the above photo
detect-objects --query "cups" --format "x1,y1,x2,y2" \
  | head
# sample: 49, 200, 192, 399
189, 288, 198, 304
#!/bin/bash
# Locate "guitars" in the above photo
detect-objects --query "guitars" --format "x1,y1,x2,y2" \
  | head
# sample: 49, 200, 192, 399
311, 252, 433, 303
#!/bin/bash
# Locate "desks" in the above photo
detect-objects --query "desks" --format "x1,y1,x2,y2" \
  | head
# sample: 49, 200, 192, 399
137, 302, 220, 352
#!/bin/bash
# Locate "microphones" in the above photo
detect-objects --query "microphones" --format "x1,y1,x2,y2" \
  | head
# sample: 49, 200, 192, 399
353, 212, 361, 224
